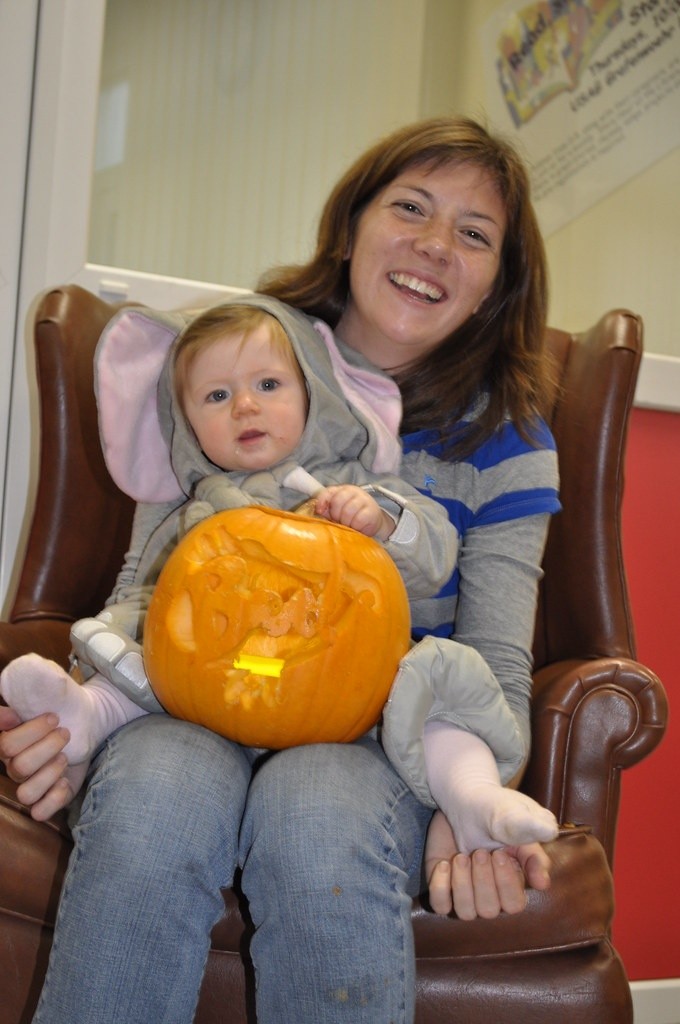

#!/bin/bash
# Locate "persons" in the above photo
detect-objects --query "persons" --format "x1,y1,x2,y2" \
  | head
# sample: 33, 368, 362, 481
0, 116, 560, 1023
0, 293, 561, 854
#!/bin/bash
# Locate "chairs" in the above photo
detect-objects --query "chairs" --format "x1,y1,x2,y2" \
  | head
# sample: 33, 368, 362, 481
0, 284, 670, 1024
